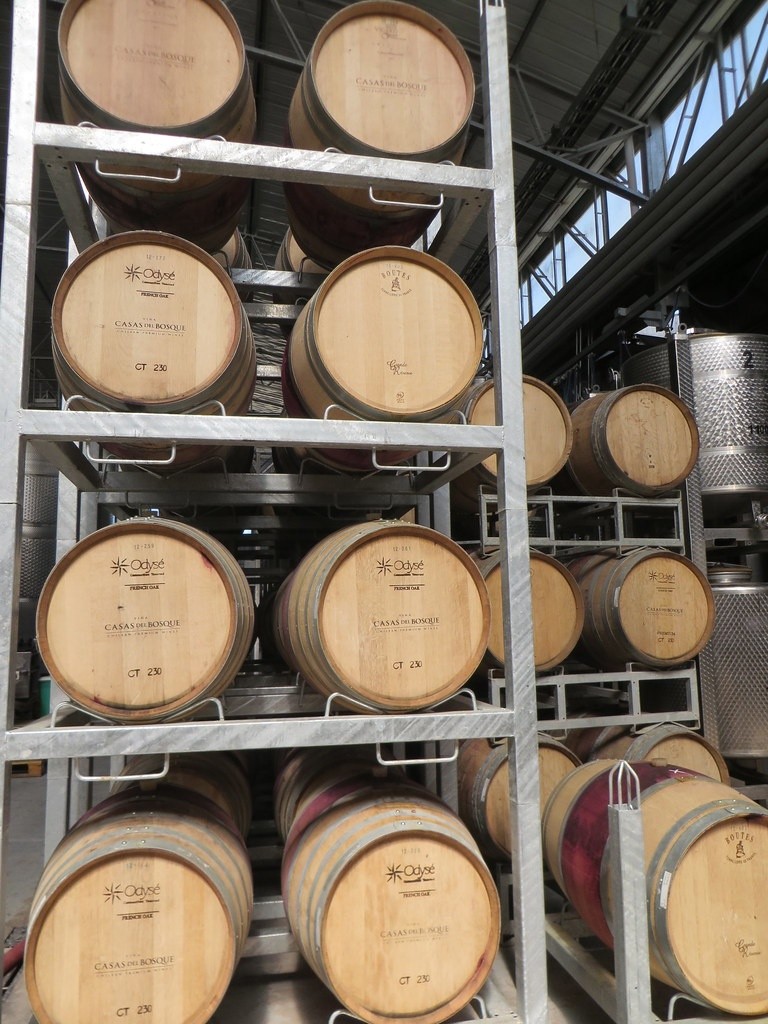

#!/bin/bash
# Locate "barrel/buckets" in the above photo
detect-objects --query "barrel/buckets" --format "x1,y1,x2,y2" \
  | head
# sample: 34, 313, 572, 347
271, 519, 714, 713
58, 1, 253, 251
49, 230, 258, 476
285, 242, 702, 493
35, 517, 255, 722
24, 755, 254, 1024
291, 5, 476, 274
273, 714, 768, 1024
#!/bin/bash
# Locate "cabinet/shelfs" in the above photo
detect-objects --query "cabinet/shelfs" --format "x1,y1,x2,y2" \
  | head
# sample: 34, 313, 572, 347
466, 484, 703, 748
0, 1, 548, 1024
